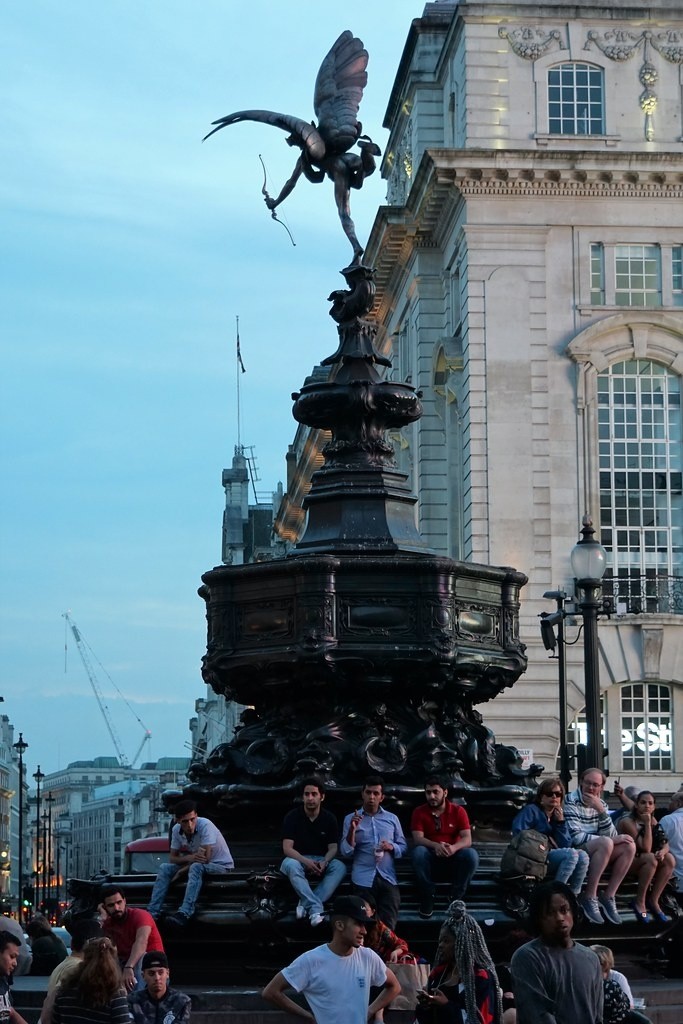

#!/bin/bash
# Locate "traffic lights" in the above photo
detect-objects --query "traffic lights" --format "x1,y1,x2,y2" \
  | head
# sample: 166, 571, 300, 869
23, 887, 30, 906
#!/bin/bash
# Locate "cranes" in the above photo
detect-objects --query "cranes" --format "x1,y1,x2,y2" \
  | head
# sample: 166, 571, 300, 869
62, 609, 153, 768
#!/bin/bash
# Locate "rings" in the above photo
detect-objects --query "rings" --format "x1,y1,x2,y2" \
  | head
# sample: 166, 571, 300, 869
560, 809, 562, 811
130, 982, 132, 985
428, 995, 433, 999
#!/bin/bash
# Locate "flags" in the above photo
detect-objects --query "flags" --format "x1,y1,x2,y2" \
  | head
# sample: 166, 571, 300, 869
237, 334, 246, 373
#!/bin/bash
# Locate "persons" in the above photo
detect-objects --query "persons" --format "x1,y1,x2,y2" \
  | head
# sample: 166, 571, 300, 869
38, 884, 191, 1024
610, 781, 676, 923
0, 931, 28, 1024
561, 767, 636, 924
262, 895, 505, 1024
652, 792, 683, 908
264, 120, 382, 267
341, 774, 408, 932
614, 780, 683, 823
495, 880, 653, 1024
145, 801, 234, 926
510, 778, 589, 898
0, 900, 68, 977
186, 700, 544, 788
280, 780, 346, 925
410, 775, 479, 919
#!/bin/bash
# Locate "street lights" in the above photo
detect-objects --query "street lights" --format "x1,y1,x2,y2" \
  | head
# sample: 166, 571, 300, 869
32, 764, 46, 911
55, 834, 60, 910
41, 808, 49, 913
63, 837, 71, 909
569, 515, 611, 773
45, 791, 55, 909
74, 841, 80, 878
98, 856, 103, 873
11, 732, 29, 925
85, 849, 92, 878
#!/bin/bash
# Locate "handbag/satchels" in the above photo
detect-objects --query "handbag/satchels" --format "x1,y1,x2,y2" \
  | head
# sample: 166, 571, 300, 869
384, 955, 431, 1009
503, 883, 536, 920
244, 896, 288, 926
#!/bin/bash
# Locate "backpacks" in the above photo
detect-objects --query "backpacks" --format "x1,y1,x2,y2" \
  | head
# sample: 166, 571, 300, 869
634, 823, 669, 858
500, 828, 550, 879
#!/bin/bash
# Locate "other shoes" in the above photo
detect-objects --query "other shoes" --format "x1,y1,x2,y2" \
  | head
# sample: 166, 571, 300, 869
418, 897, 434, 918
632, 901, 649, 924
167, 912, 189, 926
446, 893, 455, 904
648, 907, 668, 922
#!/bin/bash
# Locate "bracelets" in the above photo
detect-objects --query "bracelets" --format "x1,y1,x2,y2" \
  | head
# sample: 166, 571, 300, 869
125, 967, 134, 971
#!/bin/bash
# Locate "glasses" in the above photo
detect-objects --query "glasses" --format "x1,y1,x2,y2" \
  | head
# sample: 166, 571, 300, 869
542, 791, 562, 798
435, 816, 441, 832
582, 777, 603, 789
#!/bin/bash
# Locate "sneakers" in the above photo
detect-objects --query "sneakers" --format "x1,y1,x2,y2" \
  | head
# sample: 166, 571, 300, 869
309, 911, 326, 926
296, 899, 307, 919
597, 891, 623, 925
576, 890, 604, 924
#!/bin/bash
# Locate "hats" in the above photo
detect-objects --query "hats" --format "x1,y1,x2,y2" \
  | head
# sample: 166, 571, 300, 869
141, 951, 168, 970
320, 896, 377, 924
